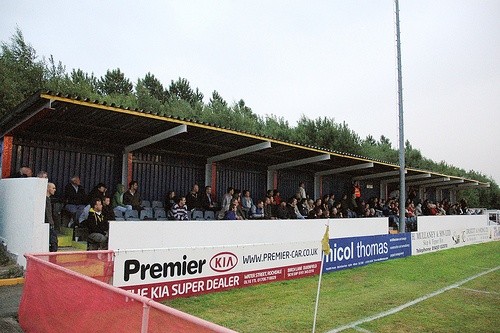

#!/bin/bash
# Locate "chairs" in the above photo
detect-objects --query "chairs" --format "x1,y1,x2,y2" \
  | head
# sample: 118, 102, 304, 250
66, 199, 219, 221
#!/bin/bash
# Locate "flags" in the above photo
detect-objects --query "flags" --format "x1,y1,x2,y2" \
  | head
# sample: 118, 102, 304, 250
322, 227, 329, 254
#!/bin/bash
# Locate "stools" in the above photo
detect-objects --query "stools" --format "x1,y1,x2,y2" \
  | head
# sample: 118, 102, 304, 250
87, 239, 102, 250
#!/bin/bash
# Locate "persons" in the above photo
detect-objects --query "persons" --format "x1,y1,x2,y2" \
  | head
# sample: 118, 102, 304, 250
165, 184, 216, 220
221, 180, 482, 231
63, 177, 146, 250
16, 166, 58, 252
